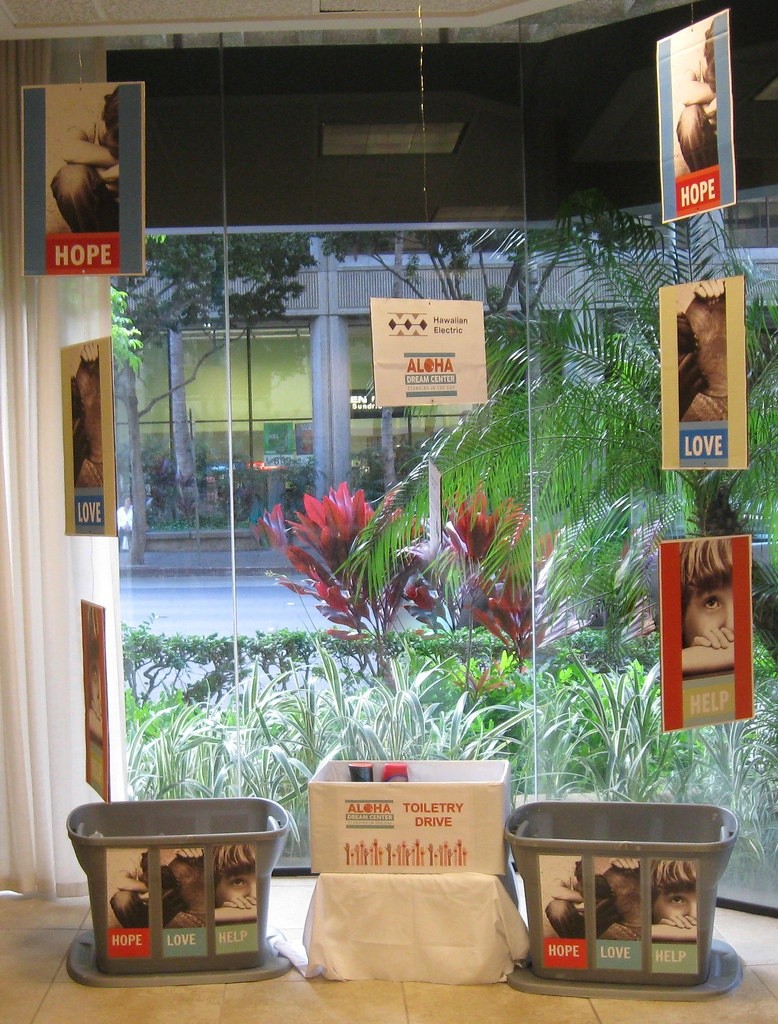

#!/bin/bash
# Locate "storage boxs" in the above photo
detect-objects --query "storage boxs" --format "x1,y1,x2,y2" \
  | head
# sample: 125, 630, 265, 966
64, 795, 292, 988
504, 800, 743, 1002
307, 759, 512, 872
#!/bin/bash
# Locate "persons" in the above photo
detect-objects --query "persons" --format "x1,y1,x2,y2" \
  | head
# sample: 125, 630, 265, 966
676, 22, 723, 177
61, 341, 104, 489
103, 844, 258, 928
539, 854, 698, 944
116, 496, 136, 552
50, 89, 122, 234
675, 535, 737, 679
